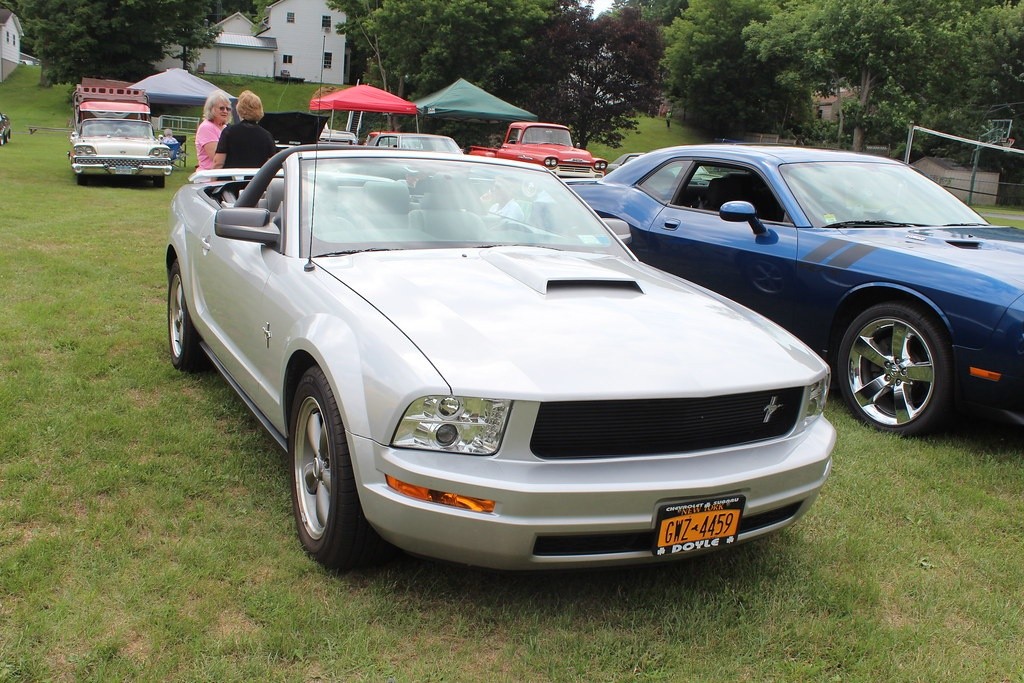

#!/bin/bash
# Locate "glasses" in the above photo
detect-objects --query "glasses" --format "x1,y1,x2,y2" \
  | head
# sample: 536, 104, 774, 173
216, 107, 231, 112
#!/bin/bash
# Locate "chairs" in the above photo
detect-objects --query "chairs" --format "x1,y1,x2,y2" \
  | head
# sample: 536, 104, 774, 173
719, 172, 747, 201
159, 142, 181, 170
166, 135, 187, 168
407, 172, 493, 241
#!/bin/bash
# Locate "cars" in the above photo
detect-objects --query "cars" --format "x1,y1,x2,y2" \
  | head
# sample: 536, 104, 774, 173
68, 118, 172, 190
364, 134, 470, 180
569, 143, 1024, 441
163, 144, 837, 572
318, 128, 359, 146
606, 153, 724, 186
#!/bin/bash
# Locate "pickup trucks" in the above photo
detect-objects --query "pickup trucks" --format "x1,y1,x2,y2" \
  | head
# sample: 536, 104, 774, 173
469, 123, 608, 181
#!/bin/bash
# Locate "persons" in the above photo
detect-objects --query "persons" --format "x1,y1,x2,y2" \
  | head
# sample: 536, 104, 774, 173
0, 113, 11, 146
490, 178, 524, 220
188, 90, 279, 184
161, 128, 178, 145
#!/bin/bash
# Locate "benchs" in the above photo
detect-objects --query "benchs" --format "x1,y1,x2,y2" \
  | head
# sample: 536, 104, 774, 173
653, 170, 724, 211
253, 178, 422, 229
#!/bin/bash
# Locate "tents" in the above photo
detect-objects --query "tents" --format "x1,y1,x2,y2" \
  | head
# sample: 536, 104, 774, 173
309, 84, 419, 144
383, 79, 538, 123
127, 68, 240, 125
